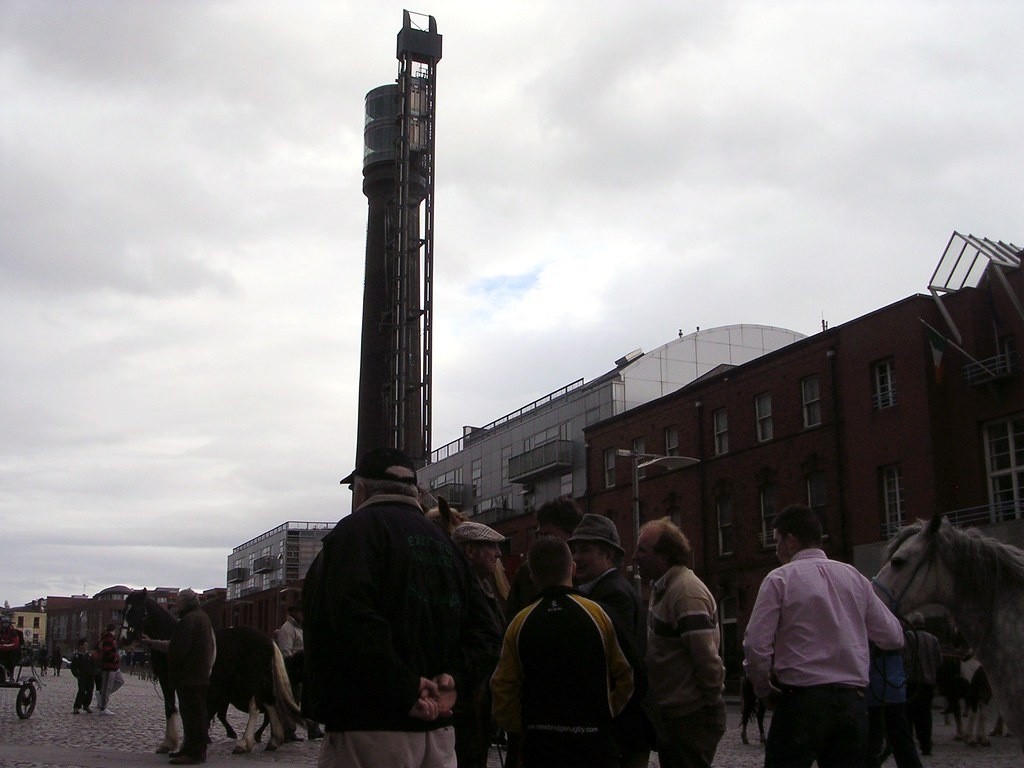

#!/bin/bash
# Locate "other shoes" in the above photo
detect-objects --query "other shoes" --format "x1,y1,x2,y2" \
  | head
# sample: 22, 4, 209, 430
921, 749, 931, 755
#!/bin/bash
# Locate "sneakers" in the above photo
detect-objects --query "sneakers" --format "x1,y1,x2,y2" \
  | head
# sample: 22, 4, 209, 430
100, 708, 115, 716
5, 677, 10, 682
308, 726, 325, 740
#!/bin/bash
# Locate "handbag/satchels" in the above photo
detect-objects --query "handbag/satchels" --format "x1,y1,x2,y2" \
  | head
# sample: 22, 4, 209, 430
92, 640, 104, 659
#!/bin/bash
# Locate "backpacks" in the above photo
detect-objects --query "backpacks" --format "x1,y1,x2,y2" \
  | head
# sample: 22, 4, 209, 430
15, 629, 24, 645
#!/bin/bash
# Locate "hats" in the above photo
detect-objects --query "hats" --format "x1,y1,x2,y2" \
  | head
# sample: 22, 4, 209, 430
907, 611, 926, 625
340, 448, 417, 486
287, 599, 303, 611
3, 618, 10, 626
566, 513, 627, 558
177, 588, 199, 602
451, 521, 506, 543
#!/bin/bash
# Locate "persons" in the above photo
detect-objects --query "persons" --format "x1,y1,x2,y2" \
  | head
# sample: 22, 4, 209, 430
632, 516, 728, 768
271, 445, 508, 768
939, 696, 1015, 737
743, 505, 943, 768
489, 497, 650, 768
0, 622, 19, 664
39, 644, 49, 676
70, 624, 124, 715
141, 590, 216, 765
127, 647, 137, 675
52, 646, 62, 676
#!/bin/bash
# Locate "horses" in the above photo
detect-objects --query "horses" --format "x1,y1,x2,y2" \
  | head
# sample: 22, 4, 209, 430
868, 514, 1024, 748
739, 672, 781, 747
118, 587, 301, 756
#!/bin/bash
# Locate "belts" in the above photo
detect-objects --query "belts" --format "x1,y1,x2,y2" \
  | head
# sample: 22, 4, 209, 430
814, 686, 867, 698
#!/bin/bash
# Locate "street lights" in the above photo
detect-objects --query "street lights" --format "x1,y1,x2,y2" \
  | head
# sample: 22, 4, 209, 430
615, 446, 700, 611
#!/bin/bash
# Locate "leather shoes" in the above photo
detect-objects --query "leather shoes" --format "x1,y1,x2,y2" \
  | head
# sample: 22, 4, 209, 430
74, 708, 80, 713
168, 749, 201, 765
82, 707, 93, 713
